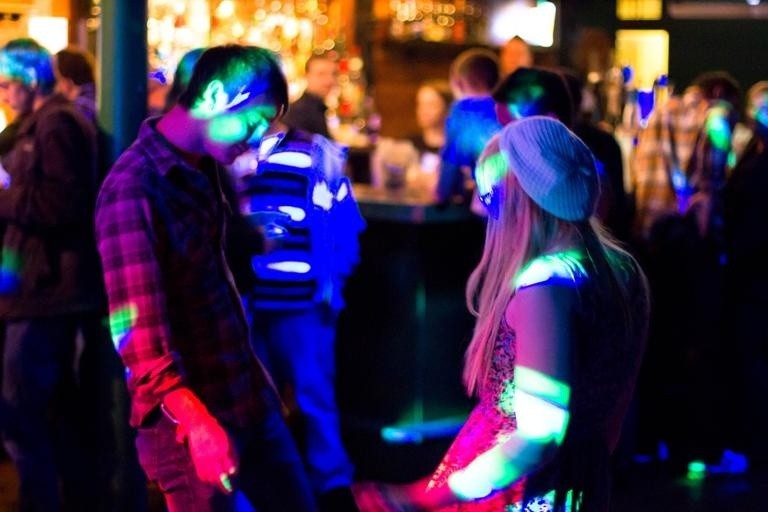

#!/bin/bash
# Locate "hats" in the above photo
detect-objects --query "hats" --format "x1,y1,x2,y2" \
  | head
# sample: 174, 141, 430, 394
498, 115, 600, 223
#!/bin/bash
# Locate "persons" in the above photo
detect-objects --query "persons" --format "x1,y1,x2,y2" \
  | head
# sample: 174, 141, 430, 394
89, 42, 321, 509
214, 104, 367, 511
420, 110, 653, 511
49, 46, 96, 119
2, 37, 121, 509
